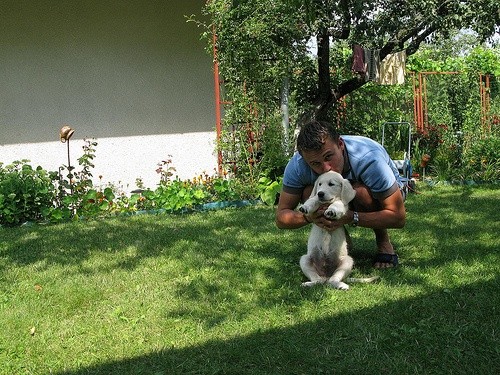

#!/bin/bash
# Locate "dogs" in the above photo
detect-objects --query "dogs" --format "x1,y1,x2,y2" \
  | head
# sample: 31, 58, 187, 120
298, 170, 381, 292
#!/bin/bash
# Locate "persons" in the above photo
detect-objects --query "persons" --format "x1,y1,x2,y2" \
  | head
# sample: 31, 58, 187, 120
276, 121, 405, 268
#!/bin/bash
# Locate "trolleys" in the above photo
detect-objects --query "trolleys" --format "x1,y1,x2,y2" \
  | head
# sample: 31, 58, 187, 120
381, 121, 416, 205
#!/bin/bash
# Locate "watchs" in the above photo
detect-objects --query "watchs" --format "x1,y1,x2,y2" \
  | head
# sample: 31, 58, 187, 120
348, 210, 359, 227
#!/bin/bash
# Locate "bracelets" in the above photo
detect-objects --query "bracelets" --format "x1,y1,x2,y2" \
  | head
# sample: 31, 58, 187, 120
302, 213, 311, 223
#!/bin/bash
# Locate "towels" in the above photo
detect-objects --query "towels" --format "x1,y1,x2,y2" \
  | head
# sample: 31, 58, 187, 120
352, 45, 406, 86
